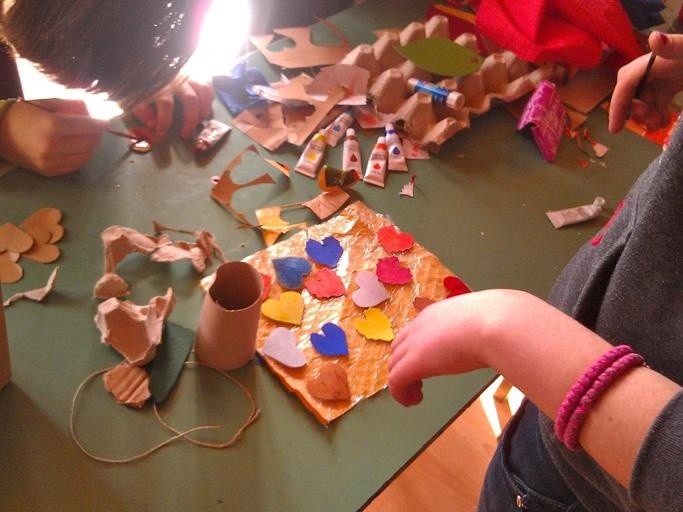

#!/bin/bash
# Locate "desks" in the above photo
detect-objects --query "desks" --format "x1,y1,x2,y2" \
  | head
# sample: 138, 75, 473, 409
0, 0, 683, 512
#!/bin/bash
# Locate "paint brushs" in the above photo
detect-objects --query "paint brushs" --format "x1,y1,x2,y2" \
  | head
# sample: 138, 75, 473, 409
625, 50, 657, 120
19, 97, 147, 140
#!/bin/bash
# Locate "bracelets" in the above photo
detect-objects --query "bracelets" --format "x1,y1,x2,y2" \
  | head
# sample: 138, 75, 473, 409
553, 344, 645, 452
0, 97, 19, 121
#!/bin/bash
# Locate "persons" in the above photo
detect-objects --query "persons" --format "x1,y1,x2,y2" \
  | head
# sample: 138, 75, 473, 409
0, 0, 214, 177
386, 31, 683, 512
